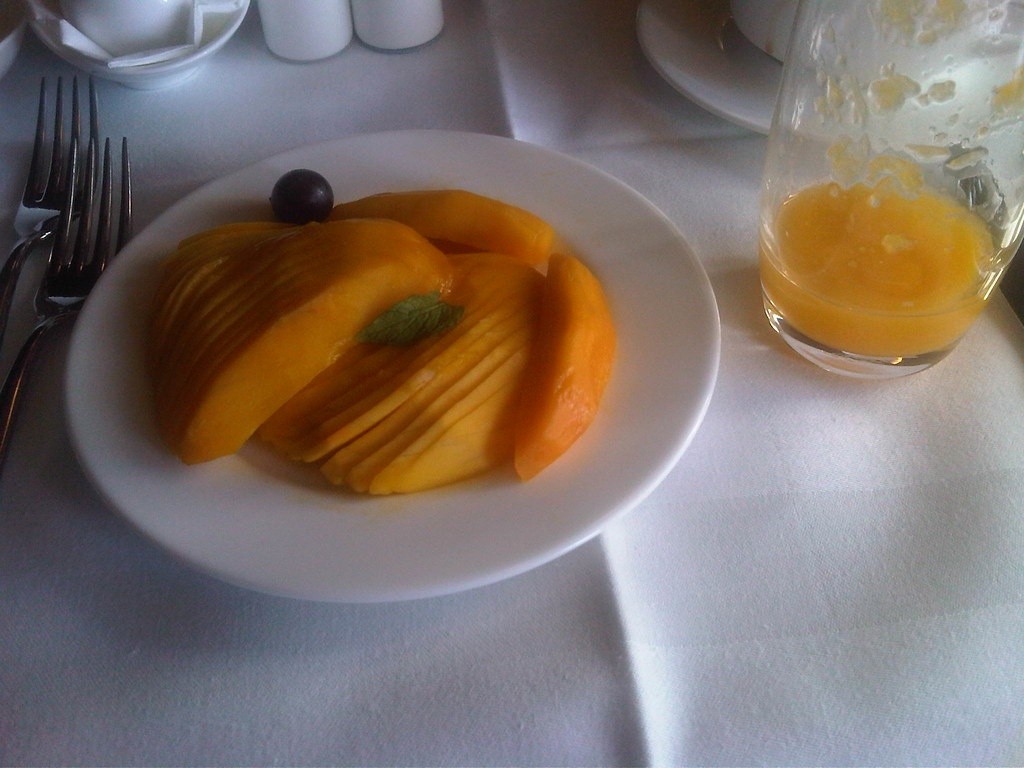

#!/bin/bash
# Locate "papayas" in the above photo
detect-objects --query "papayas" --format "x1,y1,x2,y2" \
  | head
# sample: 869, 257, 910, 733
141, 189, 614, 499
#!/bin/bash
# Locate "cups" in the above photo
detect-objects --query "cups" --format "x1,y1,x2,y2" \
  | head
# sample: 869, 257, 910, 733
731, 0, 798, 62
257, 0, 353, 63
352, 0, 445, 53
759, 0, 1024, 383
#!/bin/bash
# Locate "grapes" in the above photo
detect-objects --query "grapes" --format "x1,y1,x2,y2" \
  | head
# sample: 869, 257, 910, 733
269, 168, 334, 222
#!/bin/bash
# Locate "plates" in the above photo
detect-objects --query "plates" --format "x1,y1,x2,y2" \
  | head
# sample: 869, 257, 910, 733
636, 0, 784, 134
65, 130, 720, 601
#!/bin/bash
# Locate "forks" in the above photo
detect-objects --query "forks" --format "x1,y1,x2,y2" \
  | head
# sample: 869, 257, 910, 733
0, 77, 99, 345
0, 137, 133, 468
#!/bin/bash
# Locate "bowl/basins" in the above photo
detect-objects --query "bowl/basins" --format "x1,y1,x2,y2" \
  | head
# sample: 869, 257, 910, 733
0, 0, 26, 80
29, 0, 250, 89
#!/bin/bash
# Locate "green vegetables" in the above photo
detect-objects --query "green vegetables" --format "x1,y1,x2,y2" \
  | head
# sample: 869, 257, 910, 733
349, 296, 463, 342
